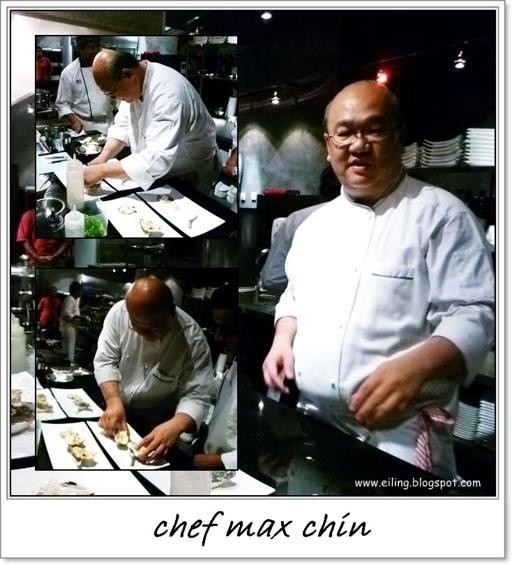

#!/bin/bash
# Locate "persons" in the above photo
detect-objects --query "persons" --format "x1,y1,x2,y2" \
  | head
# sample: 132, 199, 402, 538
93, 277, 214, 471
17, 208, 71, 268
37, 287, 61, 328
84, 50, 218, 194
35, 46, 52, 89
57, 281, 83, 362
225, 147, 237, 176
260, 166, 340, 290
123, 270, 184, 308
263, 80, 496, 474
55, 35, 115, 136
192, 284, 238, 470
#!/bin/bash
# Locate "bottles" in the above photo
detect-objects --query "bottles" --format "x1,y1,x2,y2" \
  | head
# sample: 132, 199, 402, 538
457, 189, 496, 232
43, 125, 72, 152
37, 88, 51, 111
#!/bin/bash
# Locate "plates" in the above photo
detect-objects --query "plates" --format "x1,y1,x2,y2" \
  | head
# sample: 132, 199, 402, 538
36, 151, 227, 239
401, 127, 496, 172
11, 417, 32, 435
227, 184, 237, 205
32, 467, 152, 497
85, 417, 171, 471
49, 386, 105, 419
40, 419, 116, 472
79, 145, 102, 155
35, 388, 67, 423
451, 396, 496, 451
135, 467, 278, 496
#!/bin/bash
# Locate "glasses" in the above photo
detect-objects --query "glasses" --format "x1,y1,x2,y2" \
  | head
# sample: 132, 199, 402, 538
127, 317, 171, 335
326, 122, 393, 148
103, 72, 125, 97
